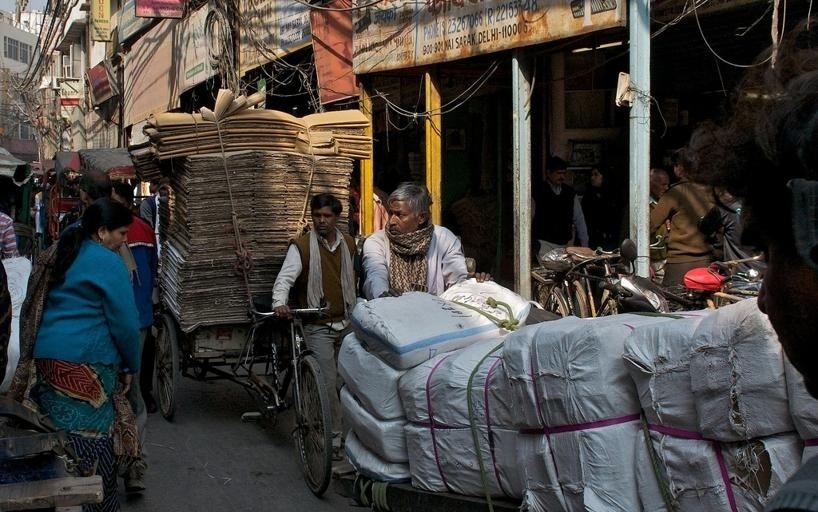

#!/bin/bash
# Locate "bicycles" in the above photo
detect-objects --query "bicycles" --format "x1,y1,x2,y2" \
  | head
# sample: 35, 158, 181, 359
530, 237, 765, 318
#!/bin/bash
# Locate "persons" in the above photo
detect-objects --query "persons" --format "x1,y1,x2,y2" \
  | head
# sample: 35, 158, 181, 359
357, 180, 498, 299
18, 196, 148, 510
0, 209, 20, 278
139, 180, 162, 227
530, 145, 753, 314
270, 191, 366, 462
56, 167, 158, 362
110, 179, 162, 415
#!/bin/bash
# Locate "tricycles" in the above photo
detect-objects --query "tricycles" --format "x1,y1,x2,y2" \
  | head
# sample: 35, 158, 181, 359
154, 298, 333, 498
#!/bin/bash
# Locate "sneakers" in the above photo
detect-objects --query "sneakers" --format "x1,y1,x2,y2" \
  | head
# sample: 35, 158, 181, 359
124, 474, 145, 491
